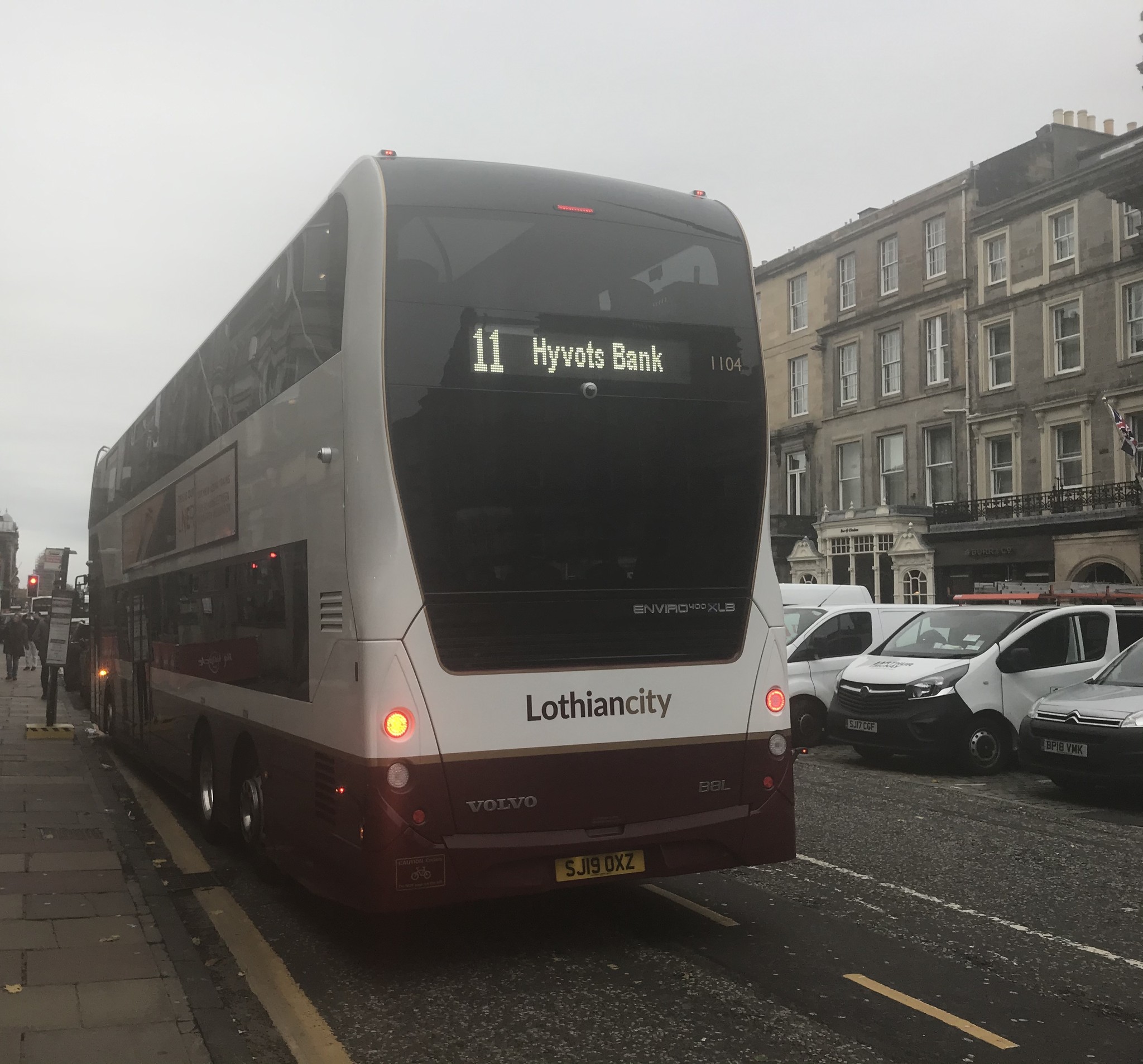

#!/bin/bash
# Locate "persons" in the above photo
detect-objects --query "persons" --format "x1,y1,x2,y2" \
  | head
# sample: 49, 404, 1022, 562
0, 611, 41, 681
31, 605, 60, 700
21, 600, 31, 613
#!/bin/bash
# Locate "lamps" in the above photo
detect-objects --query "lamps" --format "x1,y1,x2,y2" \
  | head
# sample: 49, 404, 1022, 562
809, 344, 826, 353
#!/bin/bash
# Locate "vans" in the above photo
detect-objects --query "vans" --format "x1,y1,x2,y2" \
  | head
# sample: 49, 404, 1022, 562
824, 581, 1143, 778
778, 583, 874, 644
784, 604, 980, 747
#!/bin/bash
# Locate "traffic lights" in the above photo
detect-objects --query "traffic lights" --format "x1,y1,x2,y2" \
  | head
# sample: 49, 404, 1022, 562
27, 574, 39, 598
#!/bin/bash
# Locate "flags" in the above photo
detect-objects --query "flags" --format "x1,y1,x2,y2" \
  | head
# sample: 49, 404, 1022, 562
1107, 403, 1138, 456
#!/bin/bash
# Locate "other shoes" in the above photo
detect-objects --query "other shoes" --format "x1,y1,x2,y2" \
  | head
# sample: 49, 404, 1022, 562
41, 694, 47, 699
13, 676, 17, 680
23, 667, 30, 671
5, 675, 12, 679
31, 667, 36, 671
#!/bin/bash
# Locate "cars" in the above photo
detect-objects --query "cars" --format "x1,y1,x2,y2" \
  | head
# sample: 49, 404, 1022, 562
1016, 636, 1142, 808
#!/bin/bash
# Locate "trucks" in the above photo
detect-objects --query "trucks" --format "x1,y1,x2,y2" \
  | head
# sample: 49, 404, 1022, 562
72, 595, 90, 625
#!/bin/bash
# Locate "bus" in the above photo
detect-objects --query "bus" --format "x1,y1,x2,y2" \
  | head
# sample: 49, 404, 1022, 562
72, 150, 797, 911
31, 596, 52, 620
